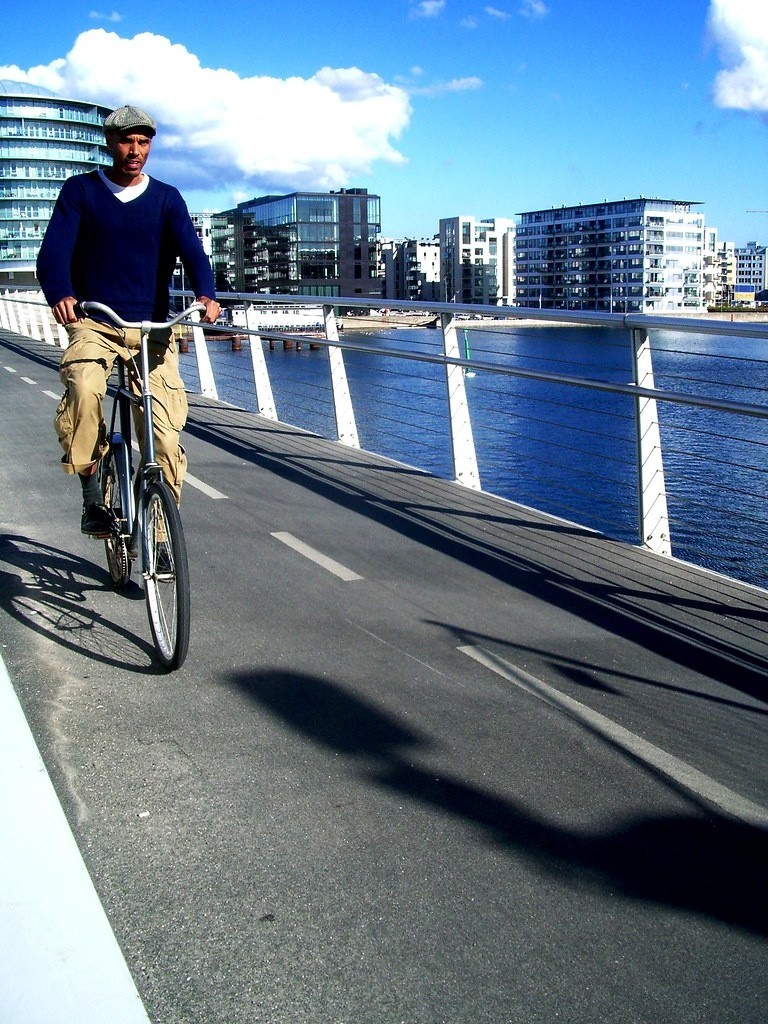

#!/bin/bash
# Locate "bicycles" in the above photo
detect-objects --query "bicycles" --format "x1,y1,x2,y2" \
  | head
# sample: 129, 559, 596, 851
70, 302, 209, 674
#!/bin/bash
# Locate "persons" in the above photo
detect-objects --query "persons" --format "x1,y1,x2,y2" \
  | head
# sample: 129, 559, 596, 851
36, 105, 223, 535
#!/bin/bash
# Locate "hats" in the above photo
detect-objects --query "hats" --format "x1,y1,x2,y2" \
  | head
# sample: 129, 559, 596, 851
104, 105, 156, 136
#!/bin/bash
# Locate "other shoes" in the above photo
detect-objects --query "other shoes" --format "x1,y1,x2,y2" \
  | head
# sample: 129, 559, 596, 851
153, 541, 174, 579
81, 501, 109, 534
